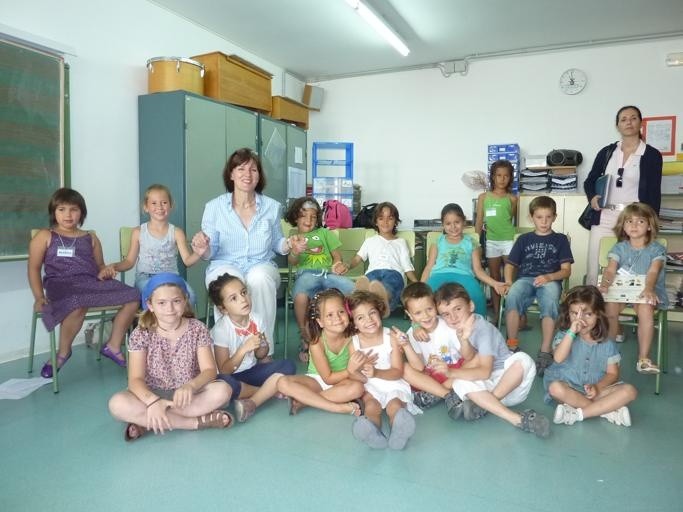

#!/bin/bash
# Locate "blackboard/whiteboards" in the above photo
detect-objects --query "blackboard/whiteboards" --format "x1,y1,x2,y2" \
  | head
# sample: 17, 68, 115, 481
0, 31, 64, 261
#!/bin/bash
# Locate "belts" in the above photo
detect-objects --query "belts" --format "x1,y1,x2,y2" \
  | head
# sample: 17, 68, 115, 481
605, 204, 625, 211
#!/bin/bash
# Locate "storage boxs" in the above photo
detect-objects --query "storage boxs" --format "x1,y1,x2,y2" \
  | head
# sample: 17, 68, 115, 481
271, 95, 311, 130
193, 53, 270, 112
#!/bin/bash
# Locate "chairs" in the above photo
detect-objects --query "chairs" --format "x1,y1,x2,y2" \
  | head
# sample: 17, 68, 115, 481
595, 237, 669, 396
494, 234, 572, 346
27, 230, 134, 394
426, 231, 480, 267
116, 225, 139, 283
277, 224, 308, 278
360, 229, 415, 307
281, 232, 366, 358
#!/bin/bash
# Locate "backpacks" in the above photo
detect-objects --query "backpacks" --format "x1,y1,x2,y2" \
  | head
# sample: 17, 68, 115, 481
321, 200, 353, 229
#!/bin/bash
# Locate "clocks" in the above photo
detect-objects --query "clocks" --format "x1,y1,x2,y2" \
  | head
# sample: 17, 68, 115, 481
560, 69, 587, 95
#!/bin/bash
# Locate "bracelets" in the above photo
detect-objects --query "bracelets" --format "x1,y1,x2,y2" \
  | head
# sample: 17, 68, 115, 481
287, 237, 293, 249
332, 261, 341, 266
290, 252, 298, 258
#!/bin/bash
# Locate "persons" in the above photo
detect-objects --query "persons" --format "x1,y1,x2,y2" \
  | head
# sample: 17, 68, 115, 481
475, 160, 528, 331
498, 196, 574, 377
579, 104, 663, 343
285, 194, 356, 364
192, 149, 300, 357
598, 203, 668, 377
98, 184, 209, 312
27, 188, 142, 377
420, 203, 507, 320
344, 202, 417, 319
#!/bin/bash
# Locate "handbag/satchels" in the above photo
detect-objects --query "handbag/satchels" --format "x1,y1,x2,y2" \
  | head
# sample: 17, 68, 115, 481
354, 203, 378, 227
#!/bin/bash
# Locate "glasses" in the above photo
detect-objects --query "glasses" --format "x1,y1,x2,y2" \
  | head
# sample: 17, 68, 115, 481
616, 168, 624, 186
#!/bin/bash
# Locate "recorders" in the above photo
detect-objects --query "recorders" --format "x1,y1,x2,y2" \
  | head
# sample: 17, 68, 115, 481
546, 148, 583, 166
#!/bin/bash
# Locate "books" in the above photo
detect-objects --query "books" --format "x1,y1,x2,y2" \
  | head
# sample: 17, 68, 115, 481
519, 168, 577, 193
595, 174, 612, 208
656, 207, 683, 312
597, 272, 657, 304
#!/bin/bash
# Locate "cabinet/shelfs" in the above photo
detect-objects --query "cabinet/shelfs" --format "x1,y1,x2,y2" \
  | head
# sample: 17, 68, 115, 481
261, 115, 310, 218
138, 90, 258, 324
512, 195, 591, 312
646, 164, 682, 321
332, 227, 368, 278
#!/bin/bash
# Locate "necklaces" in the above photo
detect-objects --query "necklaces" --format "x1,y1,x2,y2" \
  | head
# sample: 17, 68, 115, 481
233, 200, 256, 209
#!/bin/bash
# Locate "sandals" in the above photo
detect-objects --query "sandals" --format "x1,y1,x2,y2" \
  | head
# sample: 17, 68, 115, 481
125, 423, 148, 441
636, 359, 660, 374
197, 399, 256, 429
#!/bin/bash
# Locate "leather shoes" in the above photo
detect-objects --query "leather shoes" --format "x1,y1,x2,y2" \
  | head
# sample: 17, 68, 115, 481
41, 349, 72, 377
100, 345, 126, 367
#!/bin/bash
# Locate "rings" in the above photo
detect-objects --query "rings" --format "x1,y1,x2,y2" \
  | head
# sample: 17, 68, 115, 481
594, 208, 597, 210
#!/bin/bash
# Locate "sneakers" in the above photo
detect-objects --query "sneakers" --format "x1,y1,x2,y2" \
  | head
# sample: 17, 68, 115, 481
536, 350, 553, 376
348, 390, 488, 449
600, 407, 631, 427
521, 403, 583, 437
